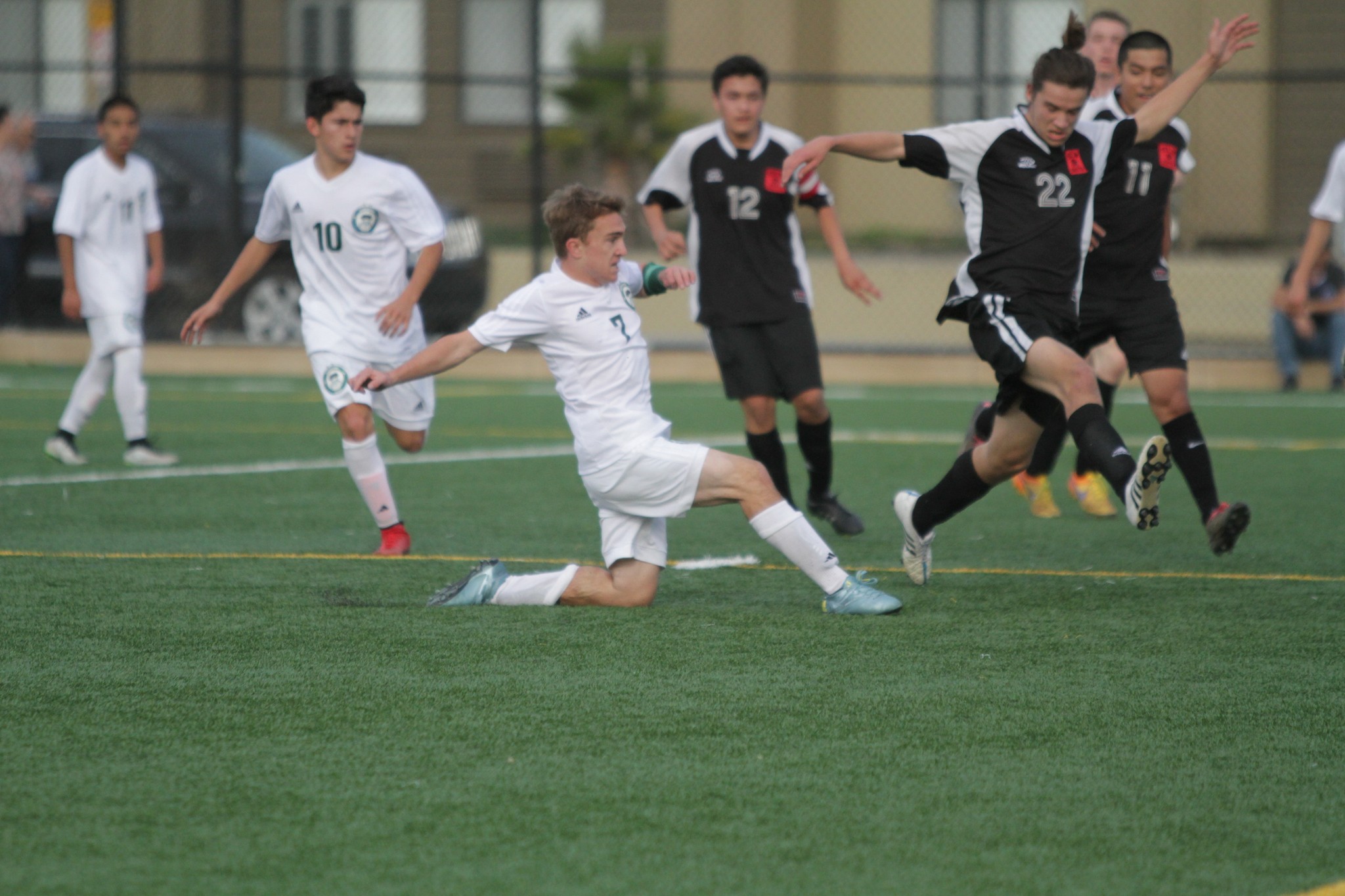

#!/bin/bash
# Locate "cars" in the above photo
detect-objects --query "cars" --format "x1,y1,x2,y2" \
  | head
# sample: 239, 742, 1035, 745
2, 108, 490, 350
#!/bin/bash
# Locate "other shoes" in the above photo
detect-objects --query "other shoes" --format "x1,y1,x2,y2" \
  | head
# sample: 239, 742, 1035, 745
121, 446, 178, 466
43, 437, 87, 467
806, 493, 865, 536
373, 533, 411, 556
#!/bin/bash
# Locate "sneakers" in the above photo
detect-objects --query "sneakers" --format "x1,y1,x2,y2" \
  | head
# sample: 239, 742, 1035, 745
427, 557, 510, 608
1205, 502, 1252, 557
1066, 471, 1118, 517
821, 571, 903, 615
1012, 469, 1059, 518
959, 403, 994, 458
891, 490, 936, 586
1124, 434, 1173, 531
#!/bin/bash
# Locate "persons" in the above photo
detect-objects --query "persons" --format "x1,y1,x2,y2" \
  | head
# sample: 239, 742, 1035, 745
779, 7, 1261, 583
176, 72, 450, 556
0, 100, 44, 320
1272, 223, 1345, 395
44, 95, 185, 468
339, 176, 904, 617
639, 52, 885, 537
961, 29, 1253, 561
1006, 10, 1145, 520
1292, 130, 1345, 311
